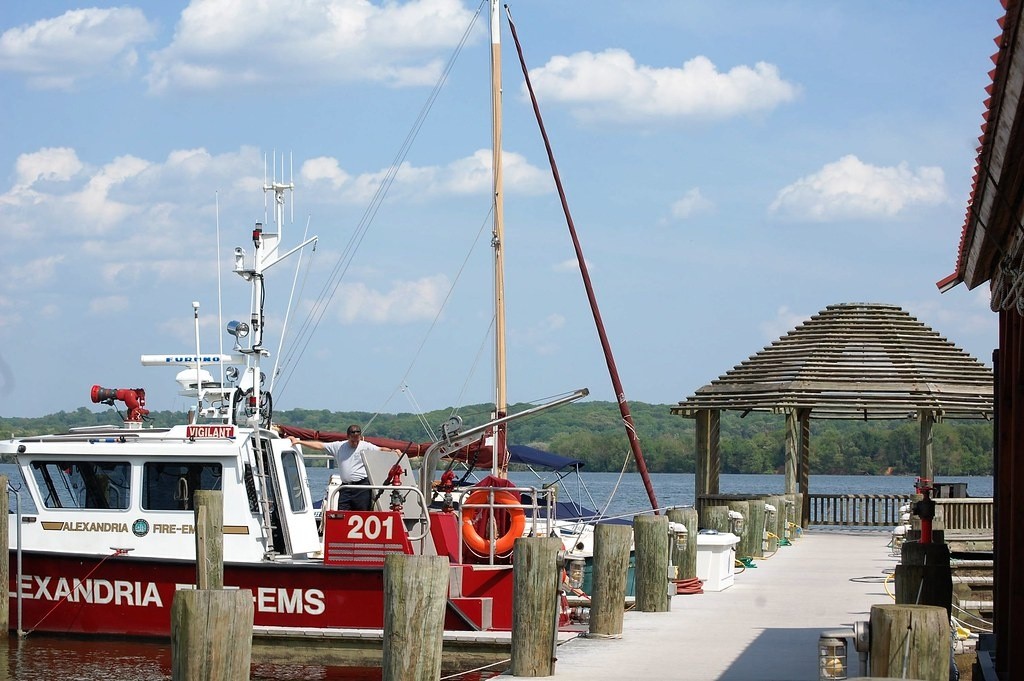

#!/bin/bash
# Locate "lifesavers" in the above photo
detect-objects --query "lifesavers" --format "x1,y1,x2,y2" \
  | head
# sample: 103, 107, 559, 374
462, 490, 526, 554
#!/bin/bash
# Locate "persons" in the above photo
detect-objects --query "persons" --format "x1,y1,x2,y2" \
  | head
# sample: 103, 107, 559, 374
287, 425, 402, 514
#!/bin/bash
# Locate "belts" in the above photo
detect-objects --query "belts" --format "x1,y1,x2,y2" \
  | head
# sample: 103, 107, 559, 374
343, 478, 369, 485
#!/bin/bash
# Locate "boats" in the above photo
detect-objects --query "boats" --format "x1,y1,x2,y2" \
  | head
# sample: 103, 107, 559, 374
418, 442, 642, 609
0, 0, 575, 652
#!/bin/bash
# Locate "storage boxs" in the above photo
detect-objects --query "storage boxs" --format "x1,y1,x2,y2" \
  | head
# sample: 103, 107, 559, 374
696, 533, 741, 591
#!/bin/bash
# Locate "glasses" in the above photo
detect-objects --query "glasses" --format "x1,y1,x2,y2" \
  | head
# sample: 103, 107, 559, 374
350, 429, 361, 433
